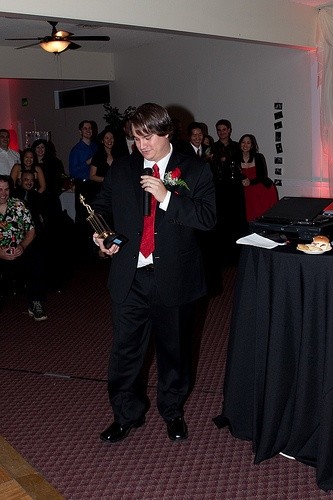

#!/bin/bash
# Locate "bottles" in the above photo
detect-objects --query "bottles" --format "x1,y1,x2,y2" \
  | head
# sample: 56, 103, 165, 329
10, 236, 17, 255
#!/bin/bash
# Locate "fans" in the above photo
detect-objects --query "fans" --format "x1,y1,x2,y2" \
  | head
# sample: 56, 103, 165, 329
4, 21, 110, 51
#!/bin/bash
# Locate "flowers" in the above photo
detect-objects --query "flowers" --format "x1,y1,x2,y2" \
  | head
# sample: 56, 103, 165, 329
162, 167, 191, 193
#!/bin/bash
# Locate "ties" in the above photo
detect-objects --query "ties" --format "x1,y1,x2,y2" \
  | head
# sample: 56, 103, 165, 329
197, 149, 200, 155
139, 163, 160, 258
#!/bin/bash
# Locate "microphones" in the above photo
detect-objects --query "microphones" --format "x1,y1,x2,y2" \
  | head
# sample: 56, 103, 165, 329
142, 167, 153, 216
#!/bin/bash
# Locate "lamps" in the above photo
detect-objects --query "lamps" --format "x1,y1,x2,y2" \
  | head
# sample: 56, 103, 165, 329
39, 40, 71, 78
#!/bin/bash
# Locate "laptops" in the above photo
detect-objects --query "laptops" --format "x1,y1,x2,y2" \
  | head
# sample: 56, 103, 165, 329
258, 196, 333, 225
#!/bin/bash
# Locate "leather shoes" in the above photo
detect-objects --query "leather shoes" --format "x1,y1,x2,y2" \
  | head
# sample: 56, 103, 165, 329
167, 416, 188, 441
100, 416, 146, 443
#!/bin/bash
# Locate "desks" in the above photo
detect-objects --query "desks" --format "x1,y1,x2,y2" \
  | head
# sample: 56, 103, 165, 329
222, 231, 333, 493
59, 188, 76, 224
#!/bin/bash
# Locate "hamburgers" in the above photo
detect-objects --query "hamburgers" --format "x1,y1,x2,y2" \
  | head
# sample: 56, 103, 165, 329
297, 235, 330, 252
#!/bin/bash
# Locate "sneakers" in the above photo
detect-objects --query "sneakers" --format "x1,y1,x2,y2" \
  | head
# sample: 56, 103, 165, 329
28, 300, 47, 320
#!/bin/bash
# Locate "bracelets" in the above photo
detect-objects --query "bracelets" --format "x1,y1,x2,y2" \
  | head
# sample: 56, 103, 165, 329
20, 245, 25, 251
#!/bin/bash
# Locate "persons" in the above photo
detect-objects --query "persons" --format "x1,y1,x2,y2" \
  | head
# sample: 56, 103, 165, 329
91, 102, 219, 442
89, 126, 119, 196
0, 129, 58, 320
119, 117, 143, 165
68, 120, 101, 243
183, 120, 278, 246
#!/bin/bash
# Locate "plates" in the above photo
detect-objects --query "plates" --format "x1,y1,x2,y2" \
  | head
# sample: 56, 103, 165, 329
296, 243, 332, 255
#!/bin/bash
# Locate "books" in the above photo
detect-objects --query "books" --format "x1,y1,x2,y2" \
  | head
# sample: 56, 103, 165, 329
258, 196, 333, 227
